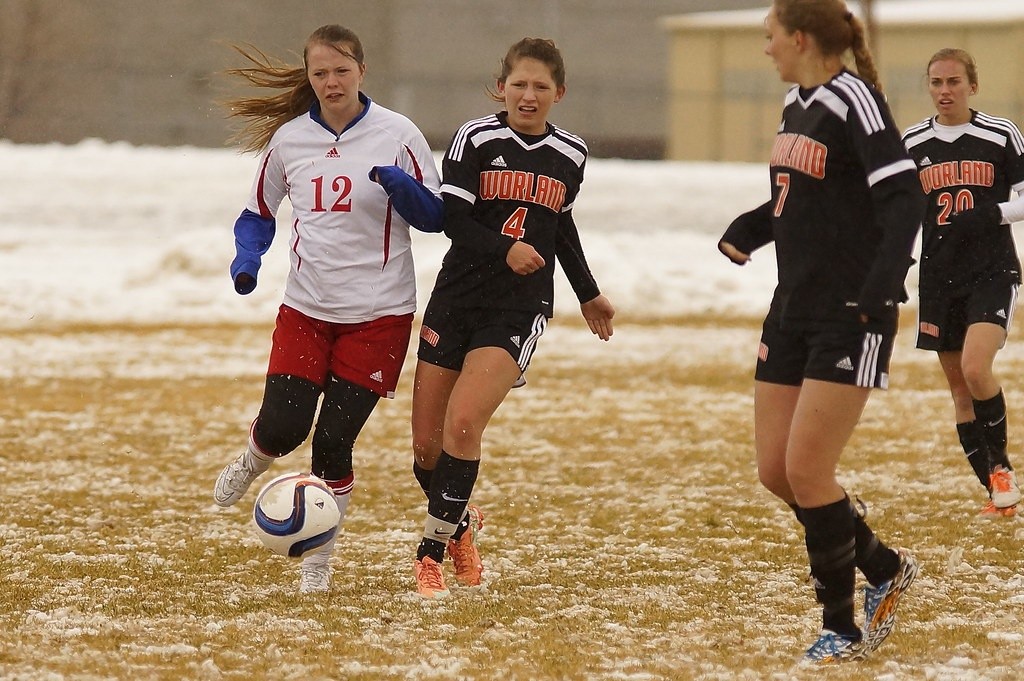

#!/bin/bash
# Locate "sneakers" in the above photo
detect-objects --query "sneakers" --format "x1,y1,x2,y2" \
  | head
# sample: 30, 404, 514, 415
298, 559, 331, 596
214, 452, 266, 505
412, 555, 450, 598
803, 629, 865, 669
983, 464, 1020, 517
861, 548, 919, 650
448, 506, 484, 586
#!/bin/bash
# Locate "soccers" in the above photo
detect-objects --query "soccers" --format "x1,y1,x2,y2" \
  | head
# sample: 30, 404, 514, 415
253, 471, 342, 560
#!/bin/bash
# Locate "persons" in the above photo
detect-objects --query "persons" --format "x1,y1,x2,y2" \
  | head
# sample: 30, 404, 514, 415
902, 48, 1024, 519
214, 23, 444, 592
718, 1, 920, 668
411, 36, 617, 599
